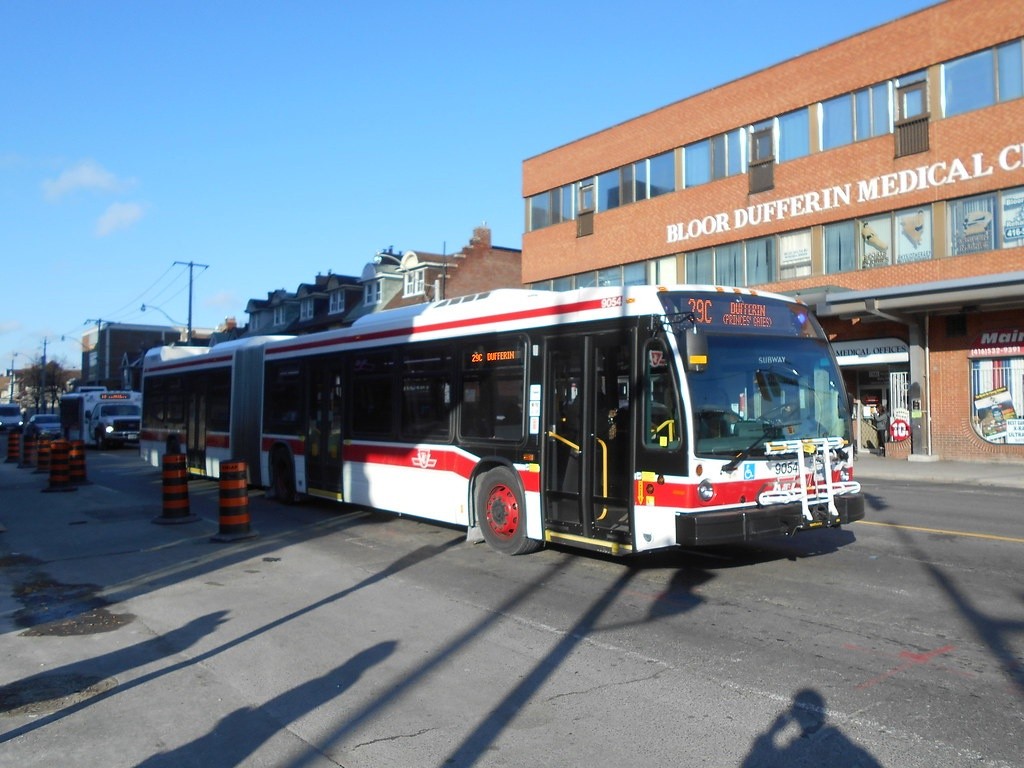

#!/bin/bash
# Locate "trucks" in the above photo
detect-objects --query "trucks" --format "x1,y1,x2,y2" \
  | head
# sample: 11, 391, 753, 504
59, 390, 143, 447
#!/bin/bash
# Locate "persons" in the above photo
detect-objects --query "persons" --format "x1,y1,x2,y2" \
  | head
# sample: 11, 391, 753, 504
693, 369, 744, 441
874, 403, 887, 455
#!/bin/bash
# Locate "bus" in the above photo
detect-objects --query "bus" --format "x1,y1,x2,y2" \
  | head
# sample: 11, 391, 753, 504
138, 280, 867, 562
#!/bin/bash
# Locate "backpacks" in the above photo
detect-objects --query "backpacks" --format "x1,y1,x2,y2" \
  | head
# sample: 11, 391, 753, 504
883, 414, 890, 432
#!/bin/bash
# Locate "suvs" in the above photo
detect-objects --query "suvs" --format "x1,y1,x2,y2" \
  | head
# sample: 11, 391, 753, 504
0, 404, 24, 433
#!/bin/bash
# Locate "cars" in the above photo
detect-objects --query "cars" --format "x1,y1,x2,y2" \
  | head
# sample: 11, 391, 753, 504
26, 414, 63, 438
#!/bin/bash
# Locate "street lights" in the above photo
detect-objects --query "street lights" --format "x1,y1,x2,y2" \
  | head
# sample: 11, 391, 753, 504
12, 351, 47, 415
139, 303, 192, 346
61, 334, 109, 385
